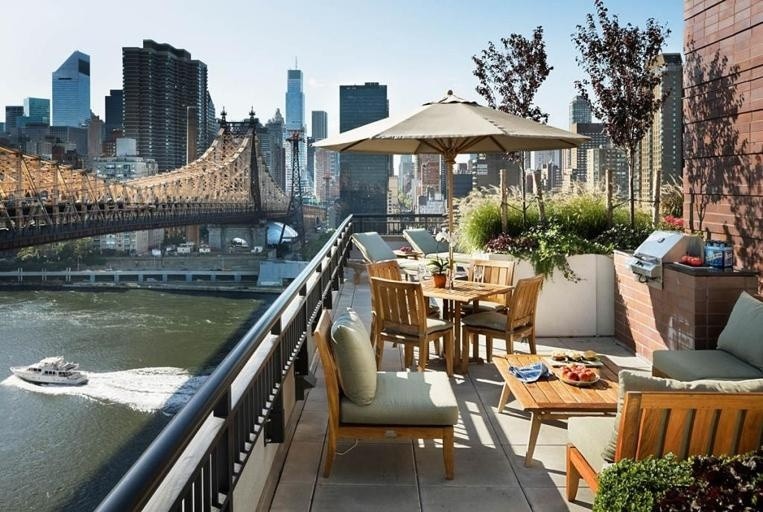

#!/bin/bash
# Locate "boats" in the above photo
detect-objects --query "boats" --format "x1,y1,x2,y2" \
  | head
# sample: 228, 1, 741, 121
10, 357, 89, 387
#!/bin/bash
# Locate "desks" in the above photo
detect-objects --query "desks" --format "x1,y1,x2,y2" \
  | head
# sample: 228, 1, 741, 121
492, 353, 623, 468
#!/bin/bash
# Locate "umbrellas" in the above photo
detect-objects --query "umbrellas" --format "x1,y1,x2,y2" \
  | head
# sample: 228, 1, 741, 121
309, 89, 593, 323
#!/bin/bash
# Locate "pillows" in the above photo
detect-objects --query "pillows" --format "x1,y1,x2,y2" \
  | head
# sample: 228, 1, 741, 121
716, 291, 763, 371
600, 371, 763, 463
329, 306, 377, 406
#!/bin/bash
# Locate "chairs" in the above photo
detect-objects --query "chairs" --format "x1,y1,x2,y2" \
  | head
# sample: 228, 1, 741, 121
403, 228, 447, 261
366, 260, 544, 377
315, 308, 458, 481
565, 368, 763, 504
350, 231, 431, 280
652, 291, 763, 382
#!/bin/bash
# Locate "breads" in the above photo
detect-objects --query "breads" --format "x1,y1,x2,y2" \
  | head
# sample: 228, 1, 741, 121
551, 349, 597, 362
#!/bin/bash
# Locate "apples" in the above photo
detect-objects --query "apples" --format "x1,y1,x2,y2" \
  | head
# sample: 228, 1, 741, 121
560, 364, 596, 382
681, 256, 701, 265
